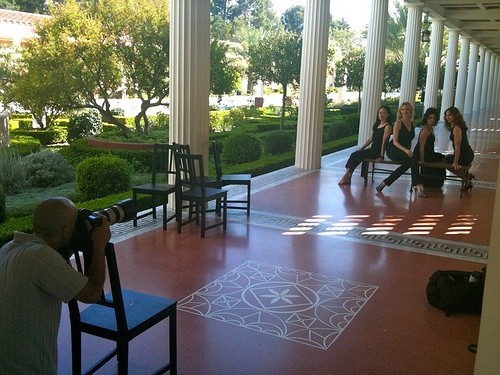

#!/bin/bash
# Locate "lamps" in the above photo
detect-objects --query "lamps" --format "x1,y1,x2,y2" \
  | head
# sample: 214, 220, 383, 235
422, 9, 431, 43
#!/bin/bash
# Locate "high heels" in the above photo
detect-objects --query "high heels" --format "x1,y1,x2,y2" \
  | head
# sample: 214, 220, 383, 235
339, 176, 352, 185
375, 182, 386, 192
464, 174, 475, 190
414, 184, 427, 198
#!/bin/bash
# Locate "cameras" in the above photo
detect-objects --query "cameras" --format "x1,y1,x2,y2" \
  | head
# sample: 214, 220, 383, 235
75, 199, 136, 237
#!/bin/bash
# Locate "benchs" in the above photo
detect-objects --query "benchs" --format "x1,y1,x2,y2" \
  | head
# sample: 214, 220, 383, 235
363, 158, 472, 198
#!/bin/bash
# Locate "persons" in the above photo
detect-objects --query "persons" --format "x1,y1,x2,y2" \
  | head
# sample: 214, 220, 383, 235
414, 108, 446, 187
444, 107, 476, 192
376, 102, 426, 197
337, 106, 392, 185
0, 197, 111, 375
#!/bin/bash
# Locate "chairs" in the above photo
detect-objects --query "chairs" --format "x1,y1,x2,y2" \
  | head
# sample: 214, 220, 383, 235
175, 143, 222, 220
174, 152, 229, 238
62, 234, 178, 375
132, 144, 179, 230
212, 139, 252, 218
